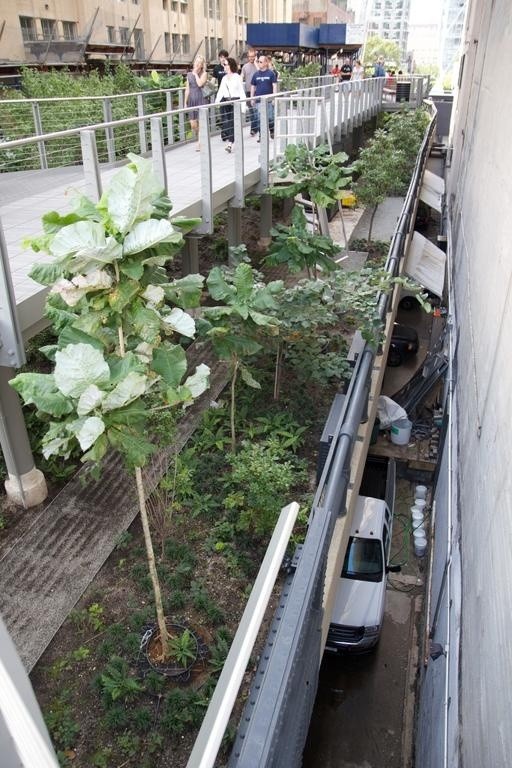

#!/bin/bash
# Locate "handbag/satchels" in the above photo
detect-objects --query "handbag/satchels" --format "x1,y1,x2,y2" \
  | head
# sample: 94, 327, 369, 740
201, 81, 218, 98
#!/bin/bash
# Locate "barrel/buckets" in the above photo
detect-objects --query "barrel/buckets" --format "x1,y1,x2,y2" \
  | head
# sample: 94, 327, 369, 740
411, 484, 428, 556
391, 420, 414, 446
395, 81, 411, 102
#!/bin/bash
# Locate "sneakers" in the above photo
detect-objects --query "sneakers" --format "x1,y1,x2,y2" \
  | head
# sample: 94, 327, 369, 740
224, 146, 232, 153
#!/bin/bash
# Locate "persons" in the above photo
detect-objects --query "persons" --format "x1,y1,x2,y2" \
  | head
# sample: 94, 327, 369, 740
338, 57, 352, 103
209, 49, 232, 88
349, 59, 364, 99
330, 59, 403, 79
250, 55, 278, 142
239, 49, 258, 136
212, 57, 248, 155
182, 54, 208, 152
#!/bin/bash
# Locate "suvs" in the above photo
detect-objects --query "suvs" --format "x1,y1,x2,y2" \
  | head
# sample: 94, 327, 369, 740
325, 494, 392, 657
